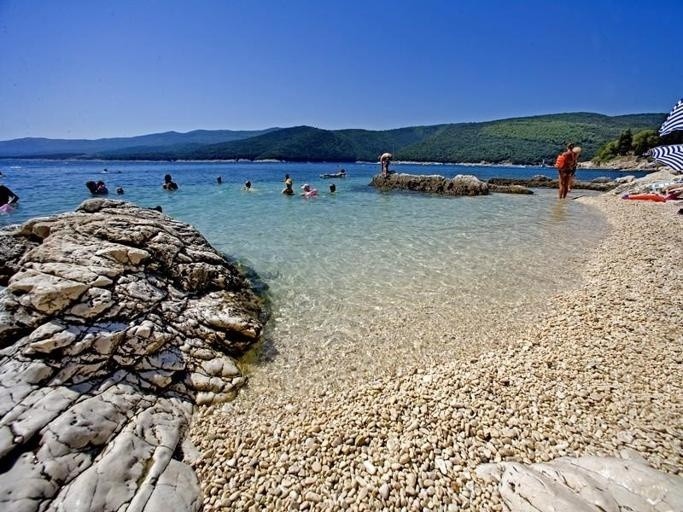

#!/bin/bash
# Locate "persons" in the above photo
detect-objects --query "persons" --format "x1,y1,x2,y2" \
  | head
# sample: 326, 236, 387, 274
557, 146, 582, 199
567, 142, 577, 192
81, 169, 341, 202
378, 152, 392, 179
0, 183, 19, 208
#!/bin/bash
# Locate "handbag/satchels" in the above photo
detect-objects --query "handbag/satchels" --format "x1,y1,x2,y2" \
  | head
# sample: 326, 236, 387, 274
555, 155, 568, 169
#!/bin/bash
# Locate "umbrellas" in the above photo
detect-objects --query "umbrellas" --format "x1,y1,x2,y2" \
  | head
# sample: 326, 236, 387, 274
649, 97, 682, 173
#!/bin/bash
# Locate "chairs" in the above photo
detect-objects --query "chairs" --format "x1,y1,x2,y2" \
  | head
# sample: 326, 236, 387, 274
626, 180, 683, 200
